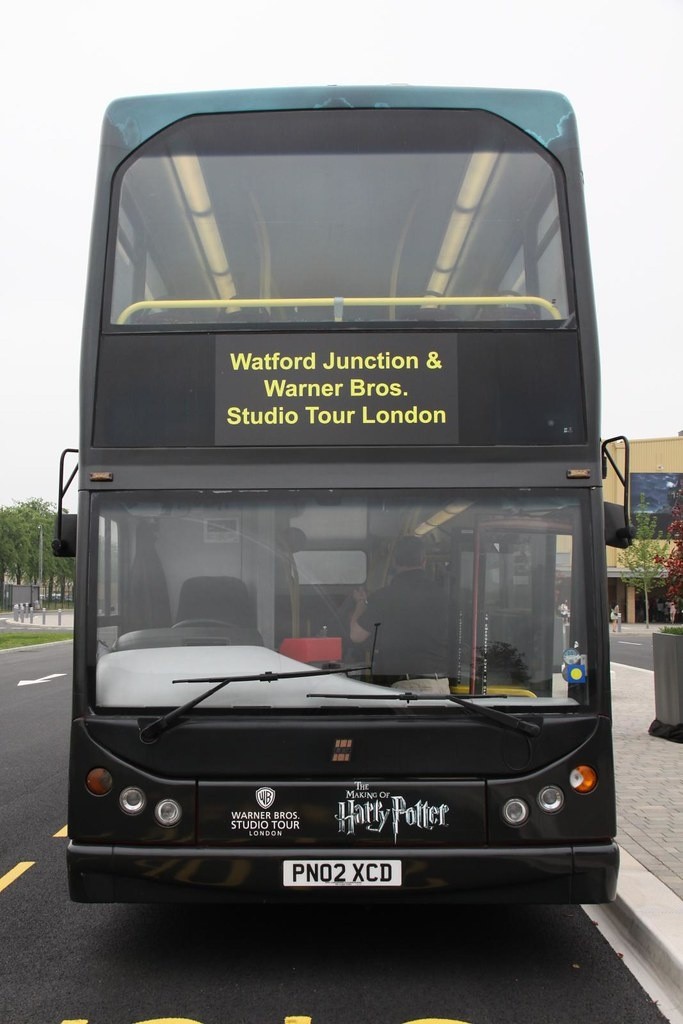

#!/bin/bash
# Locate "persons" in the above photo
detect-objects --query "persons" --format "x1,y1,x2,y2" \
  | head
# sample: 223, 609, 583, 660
349, 534, 470, 681
610, 601, 620, 633
559, 598, 570, 624
656, 597, 683, 624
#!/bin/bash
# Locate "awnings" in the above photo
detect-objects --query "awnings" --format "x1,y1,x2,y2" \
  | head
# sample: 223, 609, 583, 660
554, 560, 670, 582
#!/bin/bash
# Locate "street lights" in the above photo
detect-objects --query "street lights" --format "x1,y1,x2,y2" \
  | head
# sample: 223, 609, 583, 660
38, 525, 42, 581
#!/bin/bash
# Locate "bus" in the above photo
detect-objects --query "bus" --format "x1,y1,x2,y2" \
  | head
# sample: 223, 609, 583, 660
50, 85, 637, 906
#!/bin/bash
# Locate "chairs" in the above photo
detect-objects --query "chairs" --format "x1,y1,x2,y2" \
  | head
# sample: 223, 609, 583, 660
177, 574, 251, 645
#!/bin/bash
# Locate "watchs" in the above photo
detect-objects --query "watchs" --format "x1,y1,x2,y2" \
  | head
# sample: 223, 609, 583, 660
354, 598, 371, 607
